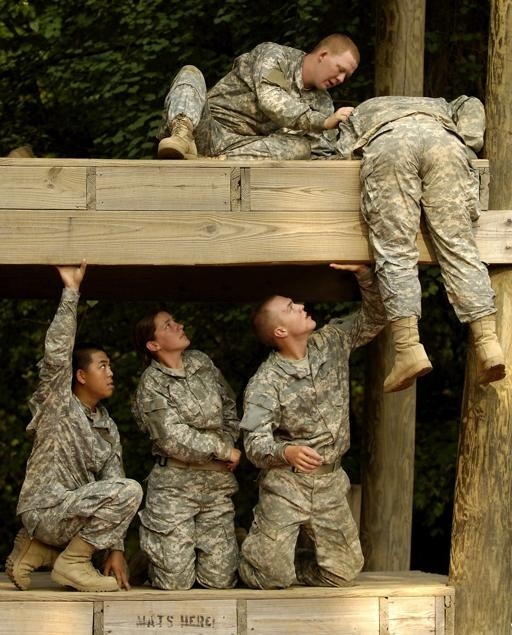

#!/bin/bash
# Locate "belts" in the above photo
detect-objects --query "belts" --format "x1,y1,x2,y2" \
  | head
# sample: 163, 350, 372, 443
283, 458, 342, 475
157, 456, 225, 473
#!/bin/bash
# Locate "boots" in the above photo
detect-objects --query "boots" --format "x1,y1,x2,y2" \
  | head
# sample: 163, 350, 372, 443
51, 533, 120, 593
383, 315, 433, 394
5, 527, 59, 591
158, 114, 199, 160
470, 313, 506, 384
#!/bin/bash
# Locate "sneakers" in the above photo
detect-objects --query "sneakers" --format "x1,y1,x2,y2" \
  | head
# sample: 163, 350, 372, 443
128, 549, 148, 587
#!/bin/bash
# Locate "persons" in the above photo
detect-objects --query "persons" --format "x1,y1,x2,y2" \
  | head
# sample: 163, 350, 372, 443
131, 310, 240, 592
336, 94, 506, 393
4, 259, 148, 591
157, 33, 361, 162
233, 263, 388, 591
